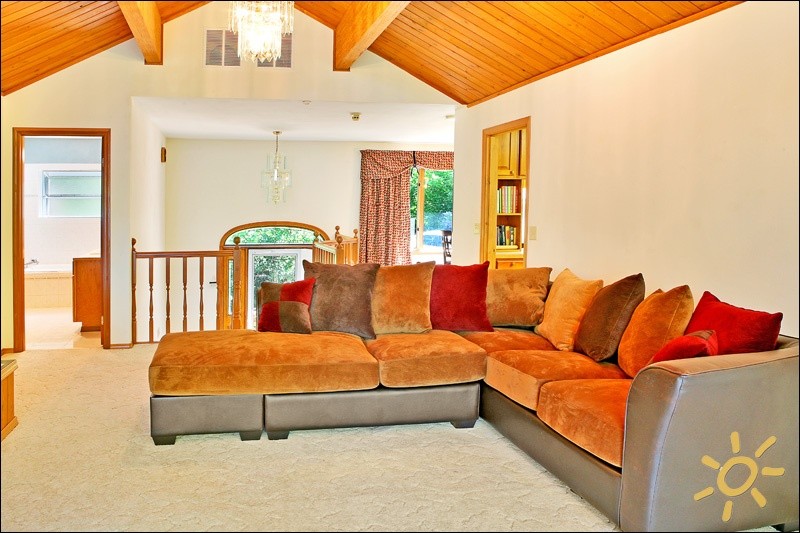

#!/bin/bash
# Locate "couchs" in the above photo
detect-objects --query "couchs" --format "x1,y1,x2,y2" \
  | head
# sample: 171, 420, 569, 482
149, 327, 800, 533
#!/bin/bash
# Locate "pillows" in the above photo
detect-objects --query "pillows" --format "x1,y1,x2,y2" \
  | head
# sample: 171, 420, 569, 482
618, 284, 694, 378
534, 268, 604, 351
300, 260, 380, 340
684, 291, 787, 355
370, 261, 437, 334
258, 277, 315, 334
576, 273, 646, 362
645, 330, 719, 366
485, 267, 553, 328
430, 260, 495, 333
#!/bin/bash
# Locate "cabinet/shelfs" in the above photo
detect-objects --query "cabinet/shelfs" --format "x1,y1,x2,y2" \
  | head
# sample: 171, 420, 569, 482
71, 256, 103, 333
493, 130, 527, 268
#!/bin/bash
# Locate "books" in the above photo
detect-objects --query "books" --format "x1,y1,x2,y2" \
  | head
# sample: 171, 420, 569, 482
497, 185, 519, 213
497, 225, 516, 245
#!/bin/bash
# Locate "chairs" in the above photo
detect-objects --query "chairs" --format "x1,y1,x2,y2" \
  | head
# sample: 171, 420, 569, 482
442, 230, 454, 264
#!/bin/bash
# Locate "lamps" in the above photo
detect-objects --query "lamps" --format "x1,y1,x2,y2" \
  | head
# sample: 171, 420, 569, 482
262, 131, 294, 205
227, 1, 295, 63
